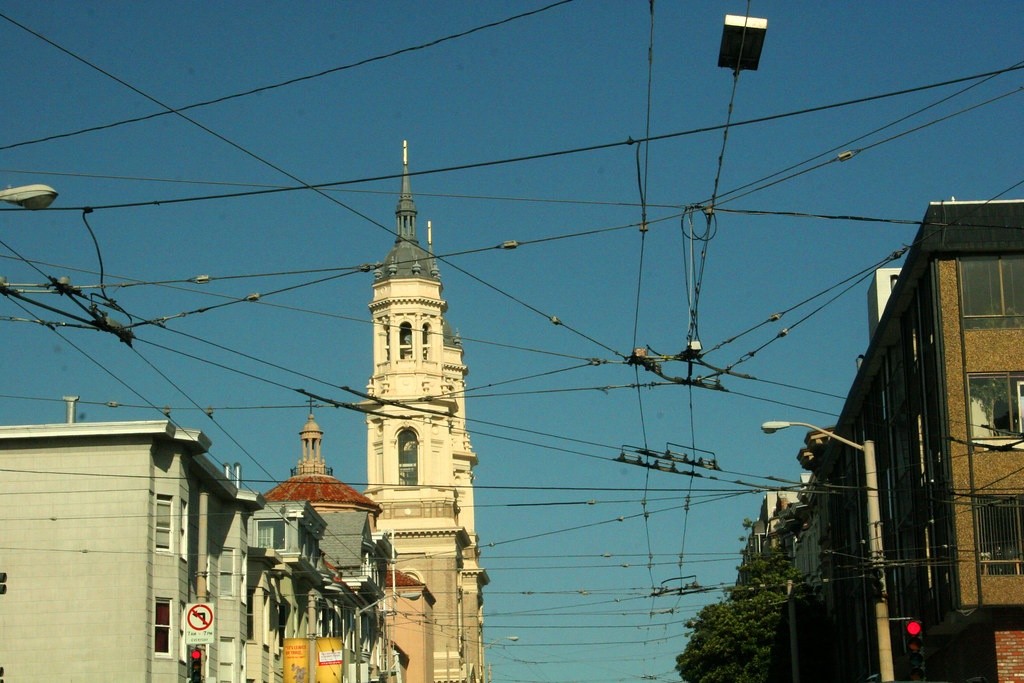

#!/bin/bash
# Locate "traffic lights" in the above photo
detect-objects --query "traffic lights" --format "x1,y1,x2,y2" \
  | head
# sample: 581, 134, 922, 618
901, 619, 927, 683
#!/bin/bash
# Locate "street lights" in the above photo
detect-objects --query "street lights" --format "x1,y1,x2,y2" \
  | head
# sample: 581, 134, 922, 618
759, 419, 895, 683
